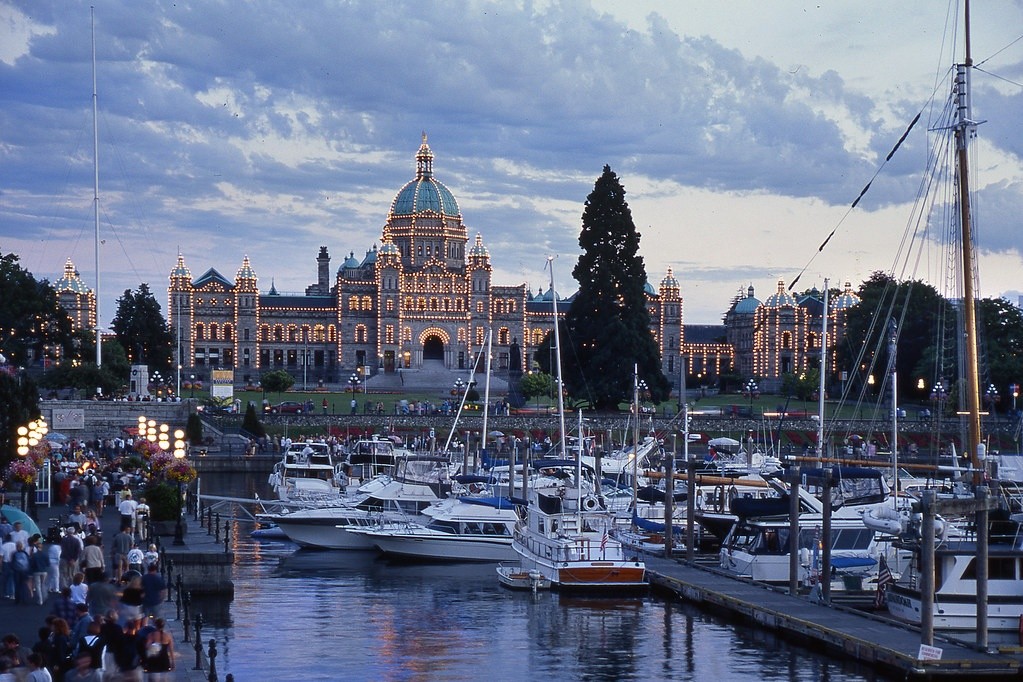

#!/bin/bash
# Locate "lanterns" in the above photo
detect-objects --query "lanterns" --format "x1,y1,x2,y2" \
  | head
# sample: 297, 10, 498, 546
17, 415, 48, 455
139, 416, 184, 459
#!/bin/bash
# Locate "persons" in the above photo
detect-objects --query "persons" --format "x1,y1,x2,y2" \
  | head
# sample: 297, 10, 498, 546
243, 434, 368, 455
905, 441, 918, 457
92, 394, 178, 402
544, 436, 551, 453
861, 441, 867, 456
0, 435, 180, 680
897, 407, 932, 422
639, 406, 656, 417
304, 398, 511, 416
417, 435, 463, 452
497, 437, 502, 453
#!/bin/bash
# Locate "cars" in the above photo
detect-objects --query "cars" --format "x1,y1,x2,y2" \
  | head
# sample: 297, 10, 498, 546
209, 406, 232, 413
264, 401, 305, 414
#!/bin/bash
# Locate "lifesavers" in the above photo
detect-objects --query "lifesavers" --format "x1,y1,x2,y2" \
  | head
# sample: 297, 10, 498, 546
583, 496, 599, 510
335, 472, 347, 485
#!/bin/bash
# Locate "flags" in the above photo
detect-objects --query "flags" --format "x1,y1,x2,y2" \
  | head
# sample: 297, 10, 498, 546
600, 523, 608, 552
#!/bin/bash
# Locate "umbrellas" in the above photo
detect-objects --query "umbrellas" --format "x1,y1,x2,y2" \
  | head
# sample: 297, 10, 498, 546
708, 437, 740, 457
490, 429, 504, 437
0, 505, 43, 547
847, 435, 863, 439
43, 431, 68, 440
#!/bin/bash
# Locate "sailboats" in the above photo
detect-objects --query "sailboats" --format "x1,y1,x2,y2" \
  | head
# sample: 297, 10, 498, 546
250, 0, 1022, 652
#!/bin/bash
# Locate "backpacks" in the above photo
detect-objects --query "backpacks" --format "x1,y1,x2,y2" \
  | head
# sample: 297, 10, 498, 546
14, 551, 28, 572
87, 475, 93, 483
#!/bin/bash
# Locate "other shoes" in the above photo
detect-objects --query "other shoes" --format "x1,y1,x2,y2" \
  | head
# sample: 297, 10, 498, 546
53, 590, 61, 593
4, 596, 15, 599
48, 590, 52, 593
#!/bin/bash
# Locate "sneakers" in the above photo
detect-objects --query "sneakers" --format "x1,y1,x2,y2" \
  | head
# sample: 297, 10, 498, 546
36, 600, 43, 605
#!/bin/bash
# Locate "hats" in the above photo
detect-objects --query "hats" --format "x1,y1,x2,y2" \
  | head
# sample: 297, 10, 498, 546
67, 527, 75, 533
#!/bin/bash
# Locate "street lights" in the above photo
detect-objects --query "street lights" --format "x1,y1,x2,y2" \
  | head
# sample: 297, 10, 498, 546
190, 374, 195, 397
150, 370, 164, 402
746, 379, 758, 413
453, 377, 465, 404
348, 373, 361, 400
